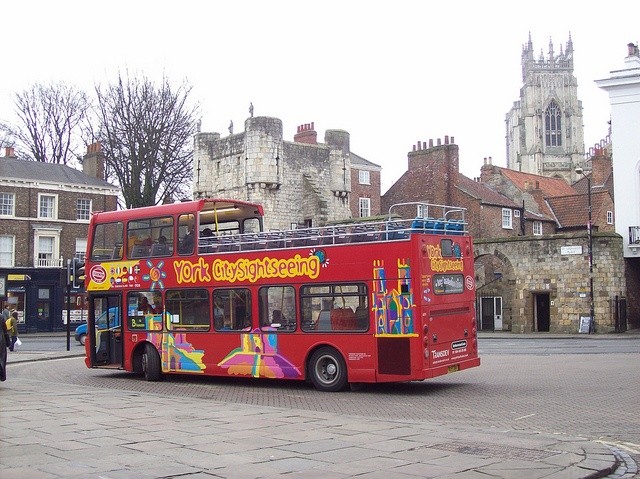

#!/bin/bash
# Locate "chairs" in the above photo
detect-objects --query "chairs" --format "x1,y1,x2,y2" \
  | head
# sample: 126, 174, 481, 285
207, 240, 219, 251
220, 239, 231, 250
255, 236, 268, 248
266, 232, 281, 249
144, 245, 150, 256
131, 245, 145, 256
291, 231, 306, 246
456, 219, 464, 233
241, 236, 255, 250
198, 240, 207, 252
316, 308, 331, 330
150, 244, 165, 255
434, 218, 446, 235
110, 245, 121, 259
335, 228, 346, 243
319, 229, 332, 244
348, 227, 363, 241
231, 238, 240, 250
308, 231, 318, 244
343, 308, 355, 330
363, 225, 374, 240
412, 216, 424, 232
425, 216, 434, 234
445, 218, 457, 235
165, 243, 174, 255
381, 225, 394, 239
281, 230, 293, 247
394, 226, 406, 239
329, 308, 344, 330
355, 305, 368, 331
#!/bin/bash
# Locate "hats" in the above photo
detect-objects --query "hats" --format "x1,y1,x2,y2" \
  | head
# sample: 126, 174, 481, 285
152, 301, 161, 305
138, 297, 148, 304
214, 296, 223, 301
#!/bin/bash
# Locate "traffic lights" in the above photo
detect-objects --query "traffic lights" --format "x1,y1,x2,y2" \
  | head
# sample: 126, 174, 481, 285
75, 259, 85, 287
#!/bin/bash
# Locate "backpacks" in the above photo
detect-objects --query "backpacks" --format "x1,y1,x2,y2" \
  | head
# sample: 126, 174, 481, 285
5, 318, 15, 330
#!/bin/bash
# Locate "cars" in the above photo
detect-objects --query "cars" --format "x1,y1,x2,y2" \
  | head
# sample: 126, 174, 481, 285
75, 305, 140, 346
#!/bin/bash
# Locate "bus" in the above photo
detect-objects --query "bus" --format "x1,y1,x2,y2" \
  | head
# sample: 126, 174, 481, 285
83, 197, 481, 391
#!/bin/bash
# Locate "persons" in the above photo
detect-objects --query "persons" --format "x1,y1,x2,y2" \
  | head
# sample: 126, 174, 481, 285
142, 235, 153, 246
202, 228, 218, 254
157, 235, 169, 256
0, 312, 11, 382
181, 224, 203, 254
135, 299, 156, 315
5, 312, 18, 352
2, 305, 10, 320
119, 231, 143, 258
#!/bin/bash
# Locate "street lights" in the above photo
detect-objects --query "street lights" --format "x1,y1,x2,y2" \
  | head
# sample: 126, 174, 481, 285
575, 168, 595, 334
